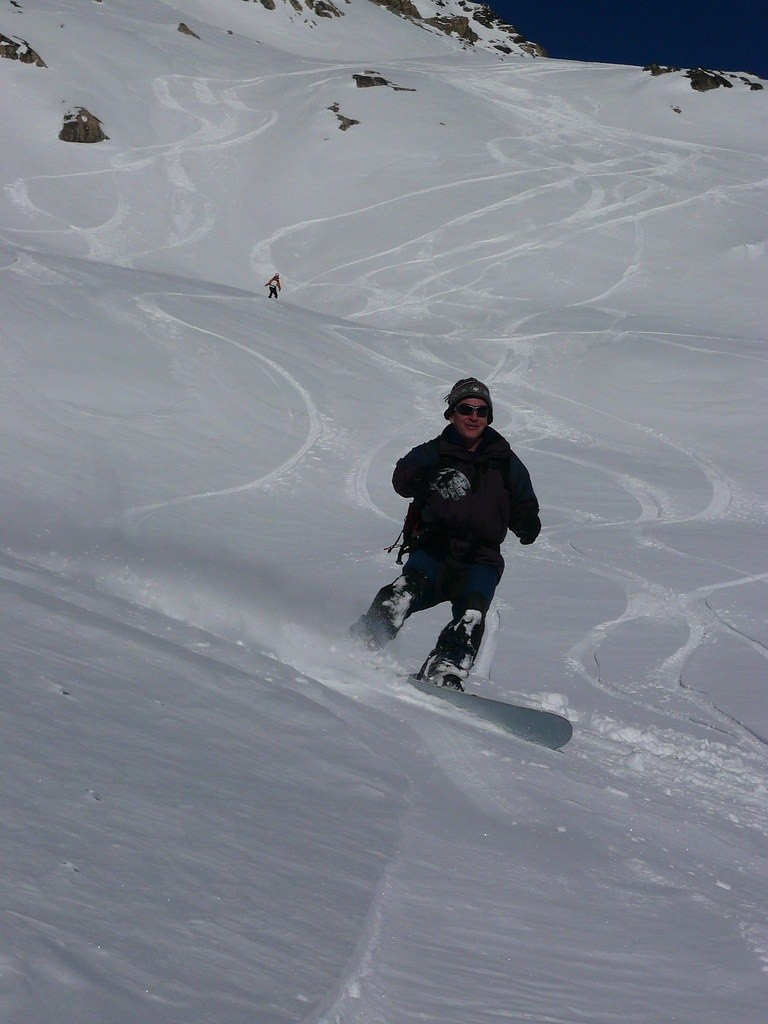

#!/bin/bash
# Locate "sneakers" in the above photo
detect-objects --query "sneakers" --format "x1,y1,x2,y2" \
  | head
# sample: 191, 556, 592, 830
417, 654, 475, 692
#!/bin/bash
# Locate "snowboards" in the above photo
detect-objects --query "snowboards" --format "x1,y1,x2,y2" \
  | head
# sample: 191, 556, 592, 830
403, 677, 572, 750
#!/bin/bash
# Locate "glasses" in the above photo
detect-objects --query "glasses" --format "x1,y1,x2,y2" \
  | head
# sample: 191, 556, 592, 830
452, 403, 490, 417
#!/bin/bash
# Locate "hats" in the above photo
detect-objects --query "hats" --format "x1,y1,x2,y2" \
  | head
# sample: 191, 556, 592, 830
443, 377, 494, 425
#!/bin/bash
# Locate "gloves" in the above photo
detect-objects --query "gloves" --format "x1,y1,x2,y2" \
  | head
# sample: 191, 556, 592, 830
424, 467, 471, 501
509, 504, 541, 544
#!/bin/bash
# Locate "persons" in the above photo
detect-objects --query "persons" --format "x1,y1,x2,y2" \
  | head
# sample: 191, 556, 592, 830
265, 273, 281, 299
351, 378, 541, 692
